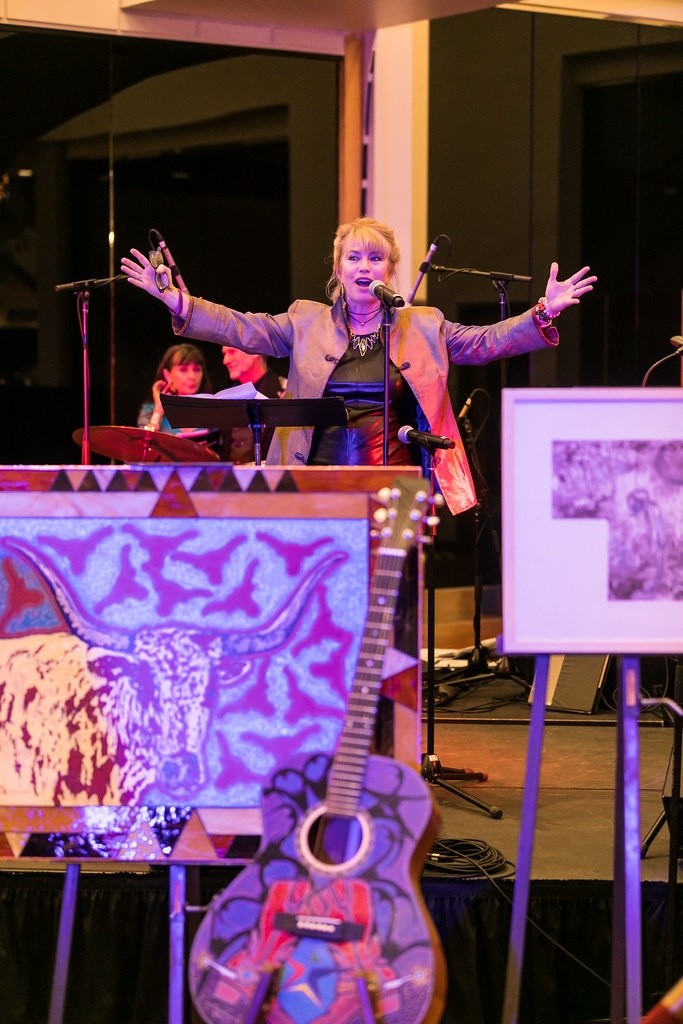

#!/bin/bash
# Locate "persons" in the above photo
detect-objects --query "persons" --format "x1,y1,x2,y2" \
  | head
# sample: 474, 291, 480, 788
139, 341, 224, 458
222, 341, 288, 460
120, 215, 599, 755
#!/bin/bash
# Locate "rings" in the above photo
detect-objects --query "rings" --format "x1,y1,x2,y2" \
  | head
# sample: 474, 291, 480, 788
140, 274, 142, 280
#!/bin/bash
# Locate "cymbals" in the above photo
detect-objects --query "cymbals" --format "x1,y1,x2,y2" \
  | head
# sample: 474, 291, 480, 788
71, 424, 222, 464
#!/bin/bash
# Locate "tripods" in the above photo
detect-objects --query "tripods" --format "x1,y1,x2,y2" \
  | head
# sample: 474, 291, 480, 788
418, 262, 533, 820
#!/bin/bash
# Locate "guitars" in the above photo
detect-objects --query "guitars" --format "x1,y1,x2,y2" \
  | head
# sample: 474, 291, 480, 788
187, 476, 450, 1024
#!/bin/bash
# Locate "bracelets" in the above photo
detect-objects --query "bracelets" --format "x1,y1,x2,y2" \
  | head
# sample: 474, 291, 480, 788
169, 288, 183, 316
536, 297, 561, 320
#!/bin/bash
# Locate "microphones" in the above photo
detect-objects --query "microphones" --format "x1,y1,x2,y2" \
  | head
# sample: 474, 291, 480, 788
397, 425, 456, 450
157, 231, 190, 296
456, 398, 472, 423
368, 280, 405, 307
406, 243, 437, 304
